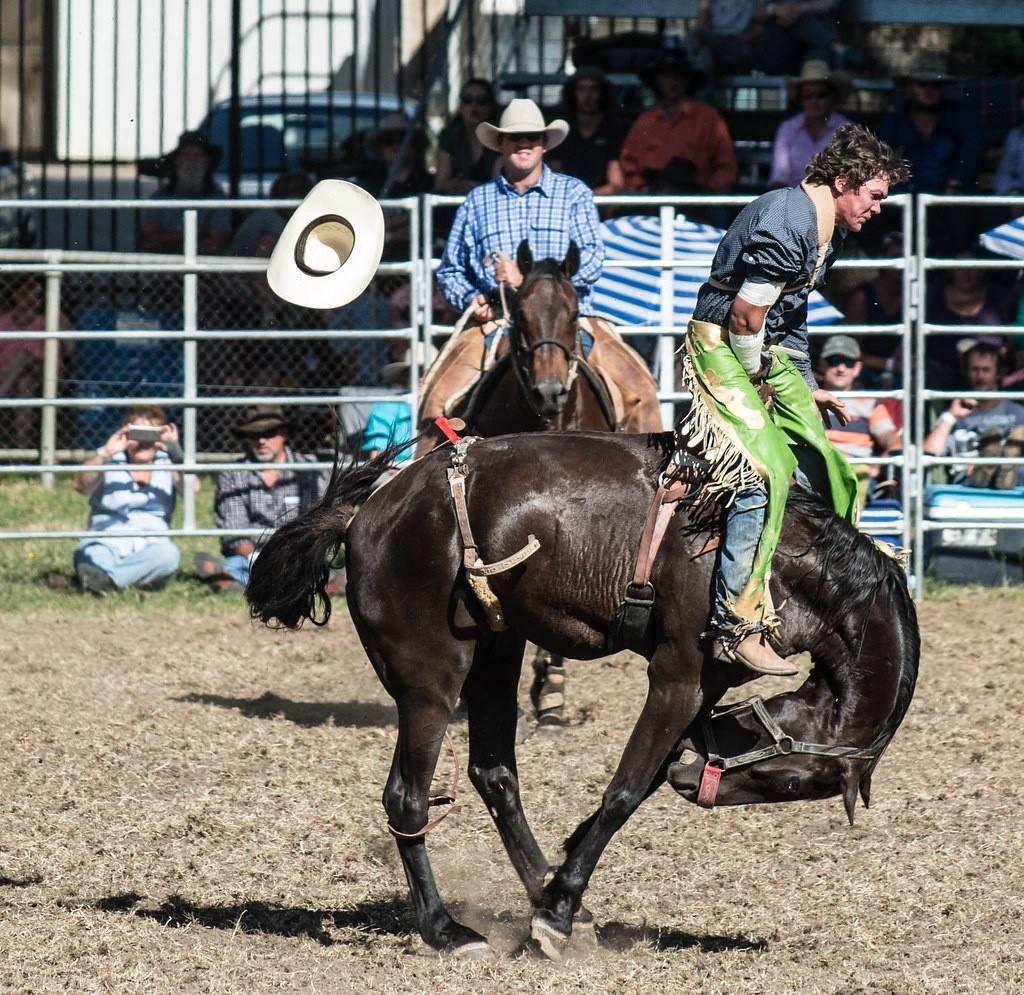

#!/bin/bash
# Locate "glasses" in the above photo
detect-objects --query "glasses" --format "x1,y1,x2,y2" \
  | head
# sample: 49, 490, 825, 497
500, 132, 544, 143
247, 430, 283, 441
823, 354, 861, 369
914, 79, 946, 90
459, 95, 492, 106
797, 86, 833, 101
379, 133, 418, 147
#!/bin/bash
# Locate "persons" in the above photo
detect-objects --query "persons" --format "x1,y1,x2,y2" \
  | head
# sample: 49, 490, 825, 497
687, 0, 1024, 485
417, 97, 665, 461
430, 49, 741, 196
0, 279, 80, 450
139, 50, 415, 400
49, 404, 201, 597
683, 122, 911, 677
196, 405, 349, 595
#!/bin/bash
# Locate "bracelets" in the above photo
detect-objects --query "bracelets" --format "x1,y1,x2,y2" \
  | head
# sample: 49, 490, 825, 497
941, 412, 957, 428
97, 449, 108, 460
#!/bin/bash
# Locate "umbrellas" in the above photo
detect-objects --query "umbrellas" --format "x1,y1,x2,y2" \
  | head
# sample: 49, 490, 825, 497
588, 217, 845, 329
980, 215, 1023, 260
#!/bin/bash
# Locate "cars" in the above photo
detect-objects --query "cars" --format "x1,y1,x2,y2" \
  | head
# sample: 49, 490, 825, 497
0, 90, 447, 458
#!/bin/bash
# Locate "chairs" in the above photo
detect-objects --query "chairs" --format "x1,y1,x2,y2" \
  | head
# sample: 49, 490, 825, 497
335, 70, 1022, 553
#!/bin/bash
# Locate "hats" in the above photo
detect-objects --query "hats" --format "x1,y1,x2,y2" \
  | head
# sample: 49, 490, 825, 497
361, 117, 430, 162
266, 178, 385, 310
228, 405, 290, 442
165, 131, 224, 180
784, 56, 853, 109
476, 98, 570, 152
819, 335, 863, 361
636, 46, 709, 99
892, 48, 960, 87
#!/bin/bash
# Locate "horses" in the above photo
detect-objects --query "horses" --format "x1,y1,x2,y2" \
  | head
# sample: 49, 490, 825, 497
245, 429, 922, 961
435, 239, 623, 742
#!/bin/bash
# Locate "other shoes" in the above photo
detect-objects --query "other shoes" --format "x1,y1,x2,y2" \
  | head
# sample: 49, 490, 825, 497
45, 572, 81, 594
324, 573, 347, 597
842, 44, 862, 69
714, 633, 800, 675
77, 562, 113, 593
193, 551, 244, 597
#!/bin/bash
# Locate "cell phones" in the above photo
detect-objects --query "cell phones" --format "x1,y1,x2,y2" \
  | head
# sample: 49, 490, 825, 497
128, 426, 162, 442
